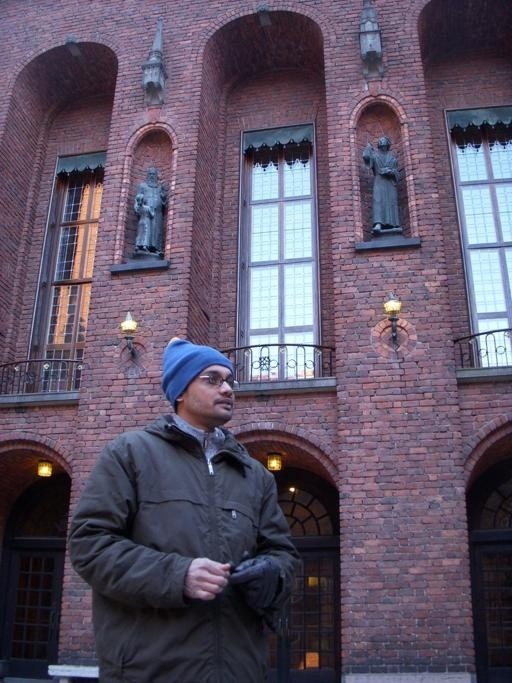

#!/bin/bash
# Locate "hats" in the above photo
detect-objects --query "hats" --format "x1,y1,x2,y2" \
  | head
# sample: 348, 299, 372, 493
160, 339, 234, 411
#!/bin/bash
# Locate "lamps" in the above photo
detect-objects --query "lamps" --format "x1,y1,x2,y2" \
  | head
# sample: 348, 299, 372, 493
37, 459, 53, 477
266, 451, 282, 472
120, 310, 139, 359
382, 290, 402, 343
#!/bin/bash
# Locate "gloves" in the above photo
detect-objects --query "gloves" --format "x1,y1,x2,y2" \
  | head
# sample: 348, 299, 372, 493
226, 555, 282, 610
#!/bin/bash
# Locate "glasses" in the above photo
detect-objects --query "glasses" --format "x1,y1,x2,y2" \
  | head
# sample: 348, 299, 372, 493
199, 375, 241, 391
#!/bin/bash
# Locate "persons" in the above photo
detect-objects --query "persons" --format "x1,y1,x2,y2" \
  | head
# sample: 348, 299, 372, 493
361, 134, 403, 237
135, 167, 167, 254
64, 334, 303, 683
